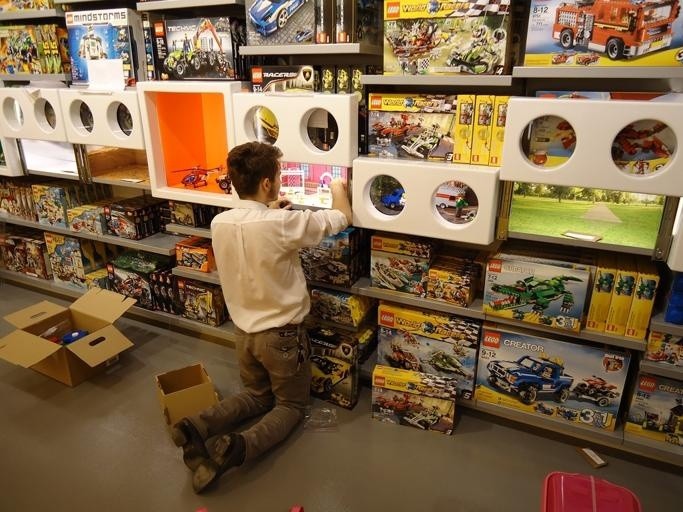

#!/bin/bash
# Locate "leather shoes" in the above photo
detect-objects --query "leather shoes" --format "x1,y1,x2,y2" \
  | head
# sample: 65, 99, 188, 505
191, 432, 245, 494
171, 416, 208, 471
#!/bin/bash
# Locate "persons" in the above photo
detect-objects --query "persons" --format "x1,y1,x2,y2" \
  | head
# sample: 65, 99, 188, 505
170, 140, 354, 497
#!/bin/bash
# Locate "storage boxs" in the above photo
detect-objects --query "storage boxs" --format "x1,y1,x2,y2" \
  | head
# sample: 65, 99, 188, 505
541, 470, 644, 512
